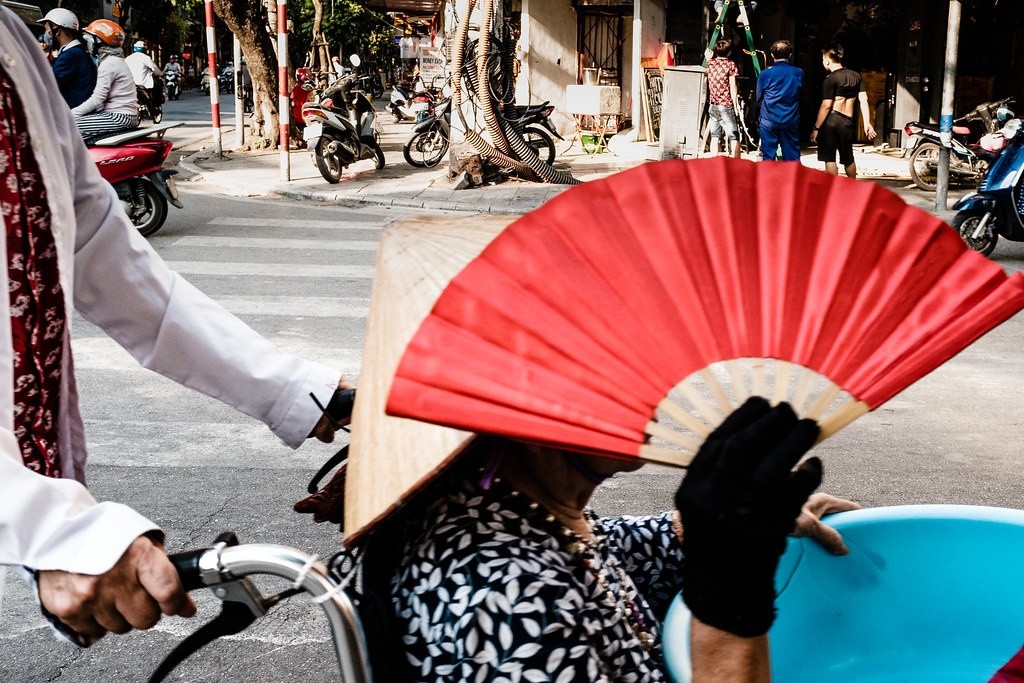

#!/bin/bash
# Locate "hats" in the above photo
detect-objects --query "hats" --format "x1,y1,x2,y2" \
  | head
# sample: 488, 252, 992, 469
169, 55, 179, 59
342, 213, 525, 543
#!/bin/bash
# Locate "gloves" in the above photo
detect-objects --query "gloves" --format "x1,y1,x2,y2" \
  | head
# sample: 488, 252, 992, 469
675, 392, 826, 640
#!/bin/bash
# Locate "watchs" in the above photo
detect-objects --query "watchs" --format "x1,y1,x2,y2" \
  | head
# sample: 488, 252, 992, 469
812, 126, 819, 131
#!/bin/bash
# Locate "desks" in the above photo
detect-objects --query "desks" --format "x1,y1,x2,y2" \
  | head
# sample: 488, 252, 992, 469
560, 113, 623, 159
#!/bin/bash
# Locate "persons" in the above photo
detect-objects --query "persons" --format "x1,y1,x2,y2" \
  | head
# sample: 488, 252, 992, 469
755, 40, 805, 163
344, 212, 887, 683
711, 0, 758, 25
704, 40, 741, 158
810, 43, 877, 180
0, 5, 358, 637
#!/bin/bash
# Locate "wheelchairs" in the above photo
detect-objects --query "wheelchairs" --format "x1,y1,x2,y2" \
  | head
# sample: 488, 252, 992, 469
147, 390, 375, 683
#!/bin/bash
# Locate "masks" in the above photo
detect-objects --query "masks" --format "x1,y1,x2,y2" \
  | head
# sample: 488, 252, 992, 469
86, 39, 97, 53
42, 26, 60, 46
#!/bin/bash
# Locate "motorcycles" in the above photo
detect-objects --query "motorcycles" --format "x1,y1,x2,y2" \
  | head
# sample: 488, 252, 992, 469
904, 96, 1016, 191
403, 95, 565, 168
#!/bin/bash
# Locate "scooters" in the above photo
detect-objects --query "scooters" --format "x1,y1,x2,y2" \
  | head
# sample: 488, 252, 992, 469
951, 108, 1023, 257
164, 70, 182, 100
384, 73, 442, 124
201, 72, 210, 95
134, 72, 166, 125
223, 71, 234, 93
87, 122, 186, 237
301, 55, 385, 185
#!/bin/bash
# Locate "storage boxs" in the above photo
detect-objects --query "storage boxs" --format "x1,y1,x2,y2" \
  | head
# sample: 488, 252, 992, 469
581, 130, 602, 154
566, 84, 621, 114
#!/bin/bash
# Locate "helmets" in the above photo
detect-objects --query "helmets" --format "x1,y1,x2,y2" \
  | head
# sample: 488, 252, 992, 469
228, 62, 233, 68
133, 40, 148, 52
216, 64, 221, 68
81, 19, 126, 48
34, 7, 80, 32
